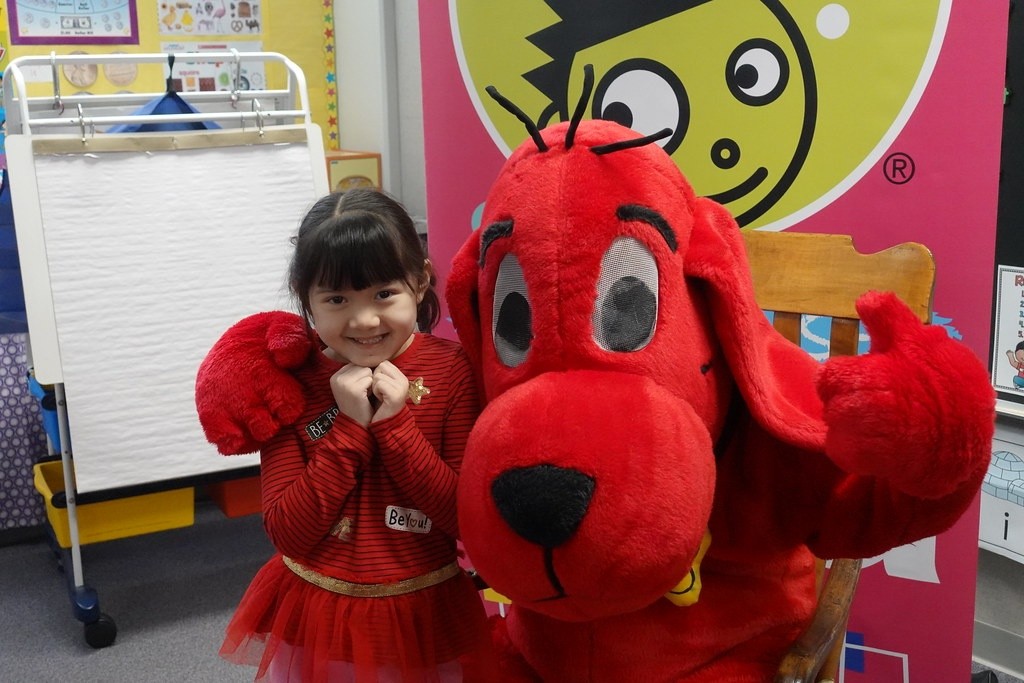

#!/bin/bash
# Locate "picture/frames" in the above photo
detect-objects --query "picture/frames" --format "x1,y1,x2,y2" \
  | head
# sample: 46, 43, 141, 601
7, 0, 140, 46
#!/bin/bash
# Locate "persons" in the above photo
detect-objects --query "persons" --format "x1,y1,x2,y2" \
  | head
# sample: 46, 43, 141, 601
218, 188, 535, 683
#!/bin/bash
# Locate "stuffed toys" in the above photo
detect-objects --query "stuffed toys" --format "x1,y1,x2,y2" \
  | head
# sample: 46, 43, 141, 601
195, 63, 997, 683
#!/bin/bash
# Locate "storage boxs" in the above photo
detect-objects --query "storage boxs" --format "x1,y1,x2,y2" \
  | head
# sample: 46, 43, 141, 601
205, 475, 266, 520
32, 458, 194, 548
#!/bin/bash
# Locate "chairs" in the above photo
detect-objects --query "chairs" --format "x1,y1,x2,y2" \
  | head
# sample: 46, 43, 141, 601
727, 232, 938, 682
